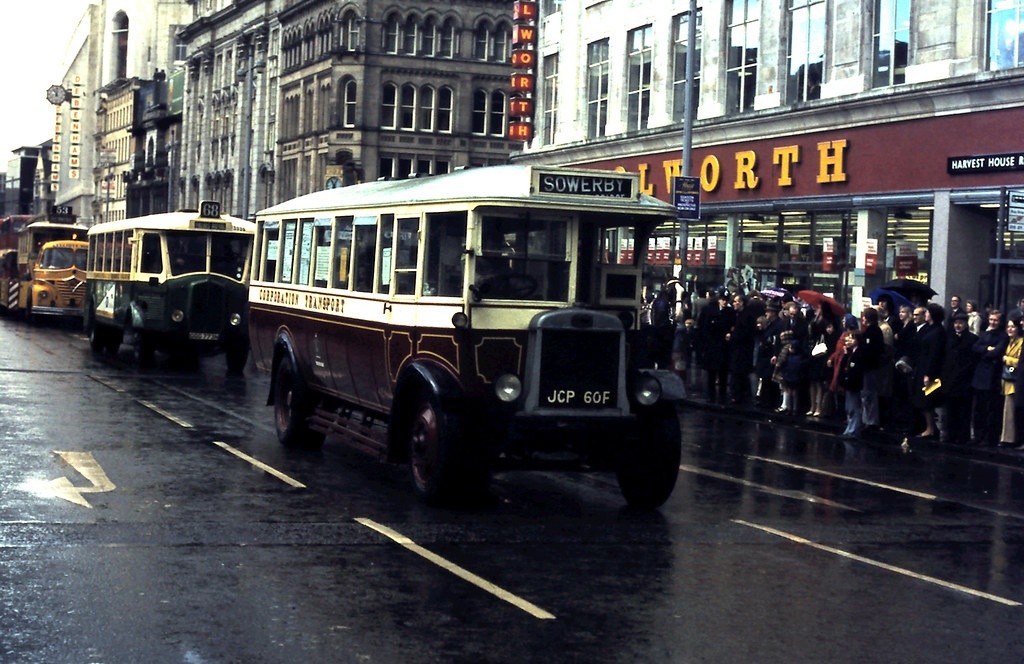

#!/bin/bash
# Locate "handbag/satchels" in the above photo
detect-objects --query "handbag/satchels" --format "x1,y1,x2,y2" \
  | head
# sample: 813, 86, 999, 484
921, 379, 942, 396
812, 334, 828, 358
1002, 364, 1017, 382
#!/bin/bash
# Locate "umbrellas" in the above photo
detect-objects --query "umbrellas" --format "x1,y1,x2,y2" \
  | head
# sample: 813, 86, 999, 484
868, 288, 916, 322
796, 290, 846, 322
881, 278, 938, 307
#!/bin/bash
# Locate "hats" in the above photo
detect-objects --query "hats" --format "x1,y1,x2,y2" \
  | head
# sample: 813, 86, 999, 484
953, 313, 969, 322
928, 304, 946, 324
719, 288, 731, 299
967, 300, 977, 311
764, 303, 780, 311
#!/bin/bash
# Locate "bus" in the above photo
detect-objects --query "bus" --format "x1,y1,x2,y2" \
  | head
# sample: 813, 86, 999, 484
248, 165, 687, 508
0, 215, 32, 309
87, 200, 255, 374
18, 205, 88, 322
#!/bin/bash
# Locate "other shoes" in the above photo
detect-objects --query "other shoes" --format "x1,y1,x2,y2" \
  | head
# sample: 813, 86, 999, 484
915, 432, 936, 438
998, 441, 1009, 446
806, 411, 813, 415
812, 411, 821, 416
775, 407, 788, 413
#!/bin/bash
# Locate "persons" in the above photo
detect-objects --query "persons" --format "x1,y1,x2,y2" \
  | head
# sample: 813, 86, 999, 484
641, 278, 1024, 450
460, 236, 523, 299
